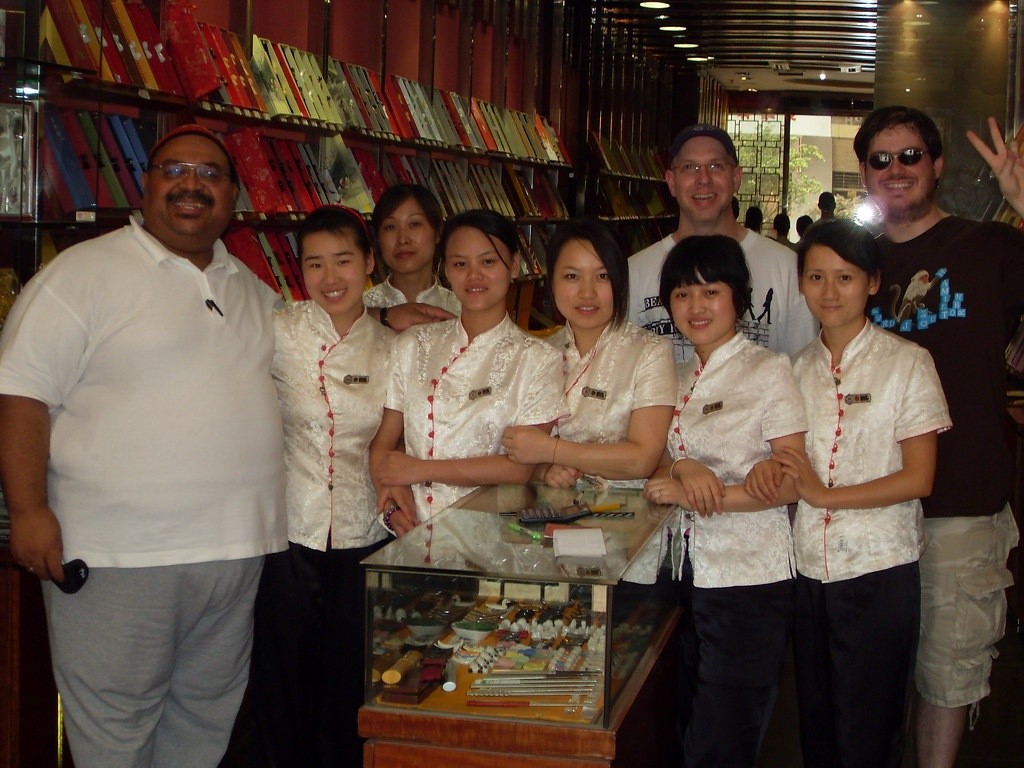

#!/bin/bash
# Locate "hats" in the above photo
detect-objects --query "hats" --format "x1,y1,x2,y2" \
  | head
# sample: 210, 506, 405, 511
667, 124, 738, 169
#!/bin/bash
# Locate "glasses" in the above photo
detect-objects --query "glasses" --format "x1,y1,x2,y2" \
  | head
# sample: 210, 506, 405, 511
868, 148, 930, 170
673, 160, 735, 175
148, 160, 233, 185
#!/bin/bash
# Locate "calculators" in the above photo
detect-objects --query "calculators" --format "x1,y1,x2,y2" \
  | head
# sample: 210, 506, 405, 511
510, 507, 591, 523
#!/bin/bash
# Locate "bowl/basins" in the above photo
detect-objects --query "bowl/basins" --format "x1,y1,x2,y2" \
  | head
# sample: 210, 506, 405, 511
451, 621, 492, 640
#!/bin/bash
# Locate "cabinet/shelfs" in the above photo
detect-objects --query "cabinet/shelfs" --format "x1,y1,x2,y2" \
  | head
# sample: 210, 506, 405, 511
355, 480, 698, 768
587, 133, 677, 222
0, 0, 574, 327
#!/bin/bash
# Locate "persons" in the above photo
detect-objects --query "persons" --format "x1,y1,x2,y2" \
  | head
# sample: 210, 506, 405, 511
369, 207, 570, 655
860, 107, 1024, 768
626, 123, 821, 362
733, 192, 836, 249
361, 183, 465, 318
647, 233, 808, 768
501, 215, 677, 491
743, 219, 953, 768
0, 121, 455, 768
219, 203, 418, 768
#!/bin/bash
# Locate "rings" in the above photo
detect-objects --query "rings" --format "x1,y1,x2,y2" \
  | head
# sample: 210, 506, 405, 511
659, 489, 663, 496
29, 566, 34, 572
559, 468, 567, 474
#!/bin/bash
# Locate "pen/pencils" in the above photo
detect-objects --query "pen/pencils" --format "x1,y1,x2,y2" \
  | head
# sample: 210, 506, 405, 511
584, 503, 626, 512
499, 512, 517, 516
573, 490, 585, 506
507, 519, 541, 540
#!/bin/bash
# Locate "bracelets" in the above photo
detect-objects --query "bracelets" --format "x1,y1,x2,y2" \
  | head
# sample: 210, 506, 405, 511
551, 438, 559, 464
383, 505, 399, 529
670, 458, 684, 479
543, 463, 552, 481
379, 307, 389, 327
